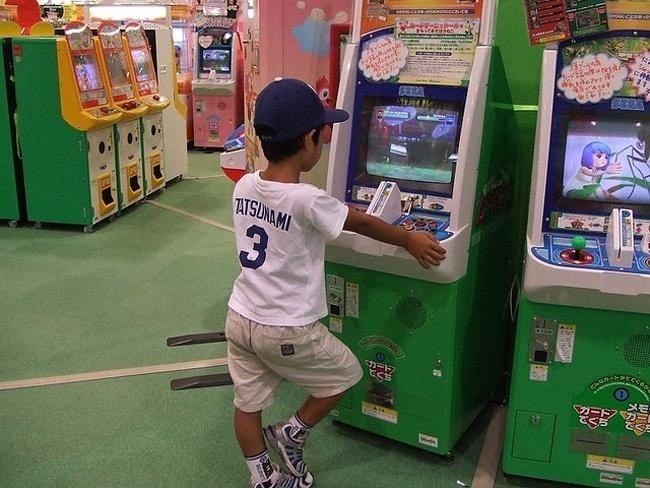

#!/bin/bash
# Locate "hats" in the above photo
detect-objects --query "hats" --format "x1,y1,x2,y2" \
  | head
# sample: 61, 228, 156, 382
253, 77, 349, 142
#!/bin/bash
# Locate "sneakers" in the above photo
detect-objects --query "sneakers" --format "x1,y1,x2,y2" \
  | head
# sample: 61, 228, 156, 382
251, 463, 314, 488
263, 419, 309, 477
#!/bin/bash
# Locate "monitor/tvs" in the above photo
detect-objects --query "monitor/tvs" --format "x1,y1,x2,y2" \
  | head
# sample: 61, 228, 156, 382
132, 51, 153, 82
564, 115, 650, 206
365, 105, 458, 184
74, 54, 103, 91
203, 49, 229, 71
106, 53, 130, 84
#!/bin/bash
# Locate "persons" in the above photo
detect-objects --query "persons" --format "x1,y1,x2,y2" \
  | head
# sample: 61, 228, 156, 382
224, 77, 446, 488
564, 141, 623, 211
